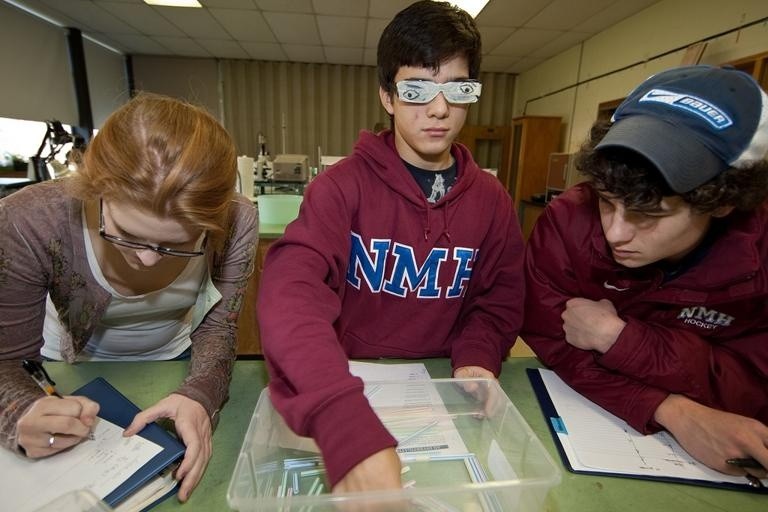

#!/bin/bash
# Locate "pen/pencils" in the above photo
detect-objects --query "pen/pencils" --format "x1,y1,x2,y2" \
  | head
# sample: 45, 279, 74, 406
22, 359, 97, 441
725, 458, 764, 469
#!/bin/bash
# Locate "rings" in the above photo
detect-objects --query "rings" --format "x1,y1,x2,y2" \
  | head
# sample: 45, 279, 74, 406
48, 434, 56, 448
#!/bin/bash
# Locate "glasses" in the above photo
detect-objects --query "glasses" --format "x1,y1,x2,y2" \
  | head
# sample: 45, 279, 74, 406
99, 198, 209, 258
387, 78, 484, 105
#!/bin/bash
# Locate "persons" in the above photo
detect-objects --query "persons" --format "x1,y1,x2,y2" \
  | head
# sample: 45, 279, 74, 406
0, 85, 261, 505
519, 63, 767, 476
259, 0, 529, 510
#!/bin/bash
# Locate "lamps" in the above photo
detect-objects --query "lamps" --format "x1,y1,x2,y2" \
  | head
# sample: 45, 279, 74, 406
30, 118, 73, 183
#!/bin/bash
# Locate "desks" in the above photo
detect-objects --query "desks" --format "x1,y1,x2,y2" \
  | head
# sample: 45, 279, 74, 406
0, 353, 767, 512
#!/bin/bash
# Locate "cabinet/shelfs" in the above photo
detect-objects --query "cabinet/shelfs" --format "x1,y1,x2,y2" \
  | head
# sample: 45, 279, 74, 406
465, 115, 585, 247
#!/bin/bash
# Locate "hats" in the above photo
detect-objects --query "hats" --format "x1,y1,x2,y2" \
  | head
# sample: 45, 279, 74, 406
590, 65, 767, 195
